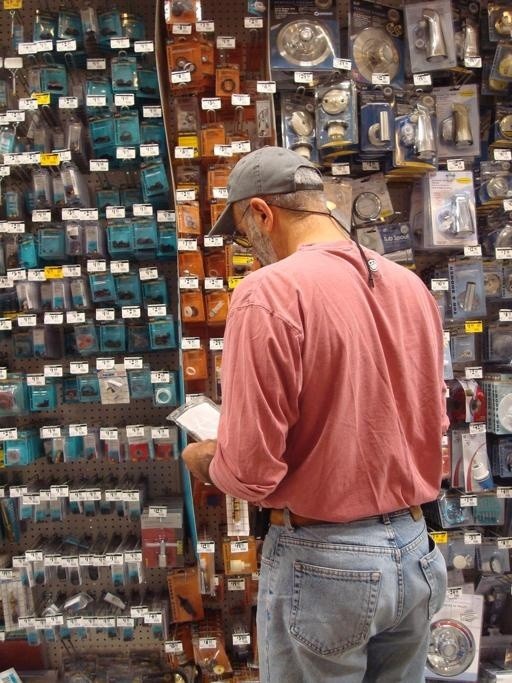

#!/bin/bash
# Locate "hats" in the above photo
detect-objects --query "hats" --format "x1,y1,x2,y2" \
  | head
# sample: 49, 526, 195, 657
207, 145, 326, 236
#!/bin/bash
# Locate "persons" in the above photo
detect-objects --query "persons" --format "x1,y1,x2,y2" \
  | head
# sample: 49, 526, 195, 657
182, 146, 446, 682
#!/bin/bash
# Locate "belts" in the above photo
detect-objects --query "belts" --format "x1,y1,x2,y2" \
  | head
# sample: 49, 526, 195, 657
269, 507, 334, 530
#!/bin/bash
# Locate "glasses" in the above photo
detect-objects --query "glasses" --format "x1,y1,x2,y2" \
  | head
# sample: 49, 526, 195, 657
230, 204, 253, 249
